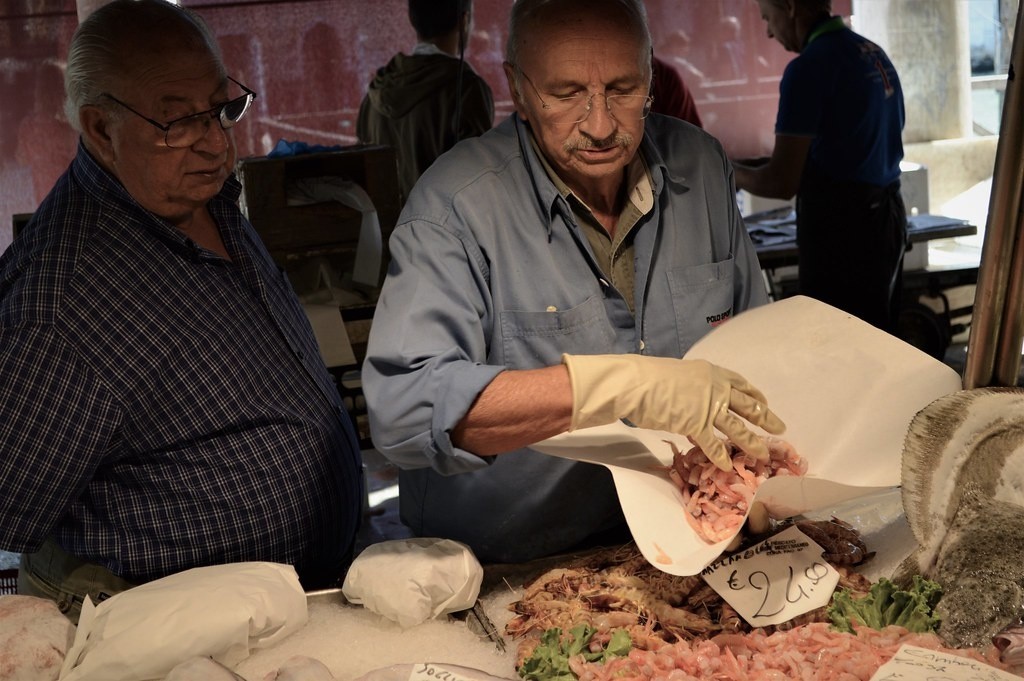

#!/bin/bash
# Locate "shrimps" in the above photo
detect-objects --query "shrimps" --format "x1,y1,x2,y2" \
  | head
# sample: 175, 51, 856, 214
500, 513, 878, 675
567, 613, 1011, 681
644, 436, 809, 545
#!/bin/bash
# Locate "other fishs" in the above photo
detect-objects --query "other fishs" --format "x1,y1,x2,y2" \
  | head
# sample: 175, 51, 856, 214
900, 387, 1023, 668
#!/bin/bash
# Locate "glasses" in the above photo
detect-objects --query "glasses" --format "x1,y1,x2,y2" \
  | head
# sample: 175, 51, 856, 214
97, 74, 256, 149
513, 46, 656, 125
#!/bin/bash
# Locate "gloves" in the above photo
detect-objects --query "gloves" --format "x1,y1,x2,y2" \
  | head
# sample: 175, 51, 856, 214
561, 352, 786, 472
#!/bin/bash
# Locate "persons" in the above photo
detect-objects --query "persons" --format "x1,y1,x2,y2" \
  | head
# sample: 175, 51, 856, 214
0, 0, 370, 628
355, 0, 908, 569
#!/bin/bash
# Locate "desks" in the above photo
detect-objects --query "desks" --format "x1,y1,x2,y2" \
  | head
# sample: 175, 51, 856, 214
755, 211, 978, 270
